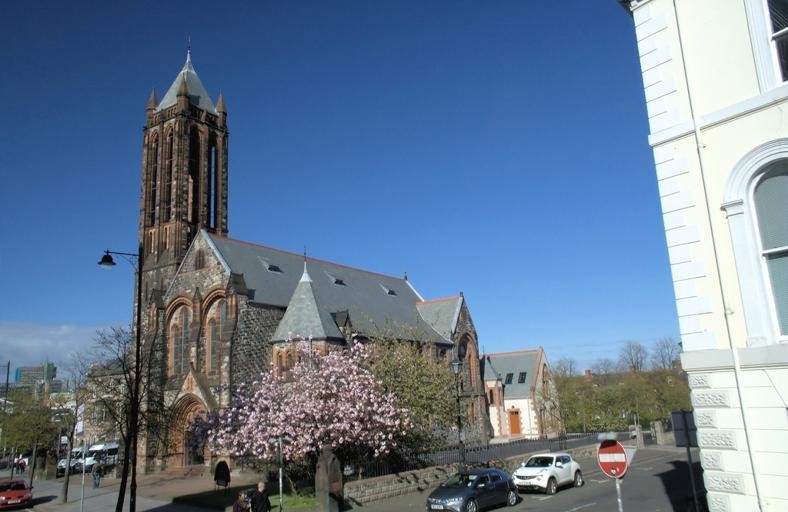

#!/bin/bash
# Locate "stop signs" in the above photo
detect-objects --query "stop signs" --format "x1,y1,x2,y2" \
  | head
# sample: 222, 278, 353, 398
595, 440, 628, 479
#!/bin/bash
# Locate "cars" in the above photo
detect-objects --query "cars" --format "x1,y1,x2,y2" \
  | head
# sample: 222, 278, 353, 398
511, 452, 583, 495
425, 466, 519, 512
0, 476, 33, 512
306, 440, 357, 477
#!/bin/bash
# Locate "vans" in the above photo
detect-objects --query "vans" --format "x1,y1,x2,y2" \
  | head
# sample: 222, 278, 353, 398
56, 442, 116, 474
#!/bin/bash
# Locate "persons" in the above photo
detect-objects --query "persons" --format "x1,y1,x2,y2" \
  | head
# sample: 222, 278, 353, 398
90, 459, 102, 489
232, 488, 251, 512
19, 458, 26, 475
251, 481, 271, 512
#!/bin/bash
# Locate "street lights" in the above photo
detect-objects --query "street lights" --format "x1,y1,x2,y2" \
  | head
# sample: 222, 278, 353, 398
449, 354, 465, 467
97, 243, 143, 512
